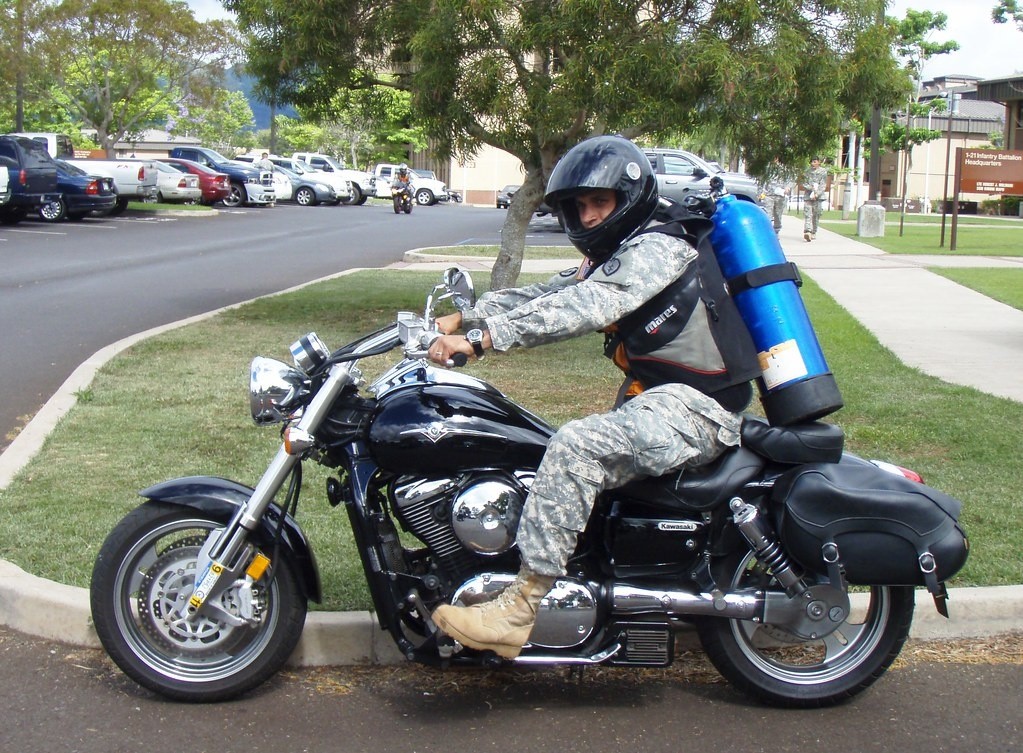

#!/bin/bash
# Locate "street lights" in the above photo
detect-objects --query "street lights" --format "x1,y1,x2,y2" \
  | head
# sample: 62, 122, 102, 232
902, 91, 948, 215
922, 103, 947, 215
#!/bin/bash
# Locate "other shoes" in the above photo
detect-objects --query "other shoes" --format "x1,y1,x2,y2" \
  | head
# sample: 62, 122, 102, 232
812, 233, 816, 240
804, 232, 812, 241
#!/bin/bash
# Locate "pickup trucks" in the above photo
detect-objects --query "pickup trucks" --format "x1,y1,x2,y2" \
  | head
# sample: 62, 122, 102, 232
10, 132, 158, 219
164, 146, 277, 208
534, 147, 761, 231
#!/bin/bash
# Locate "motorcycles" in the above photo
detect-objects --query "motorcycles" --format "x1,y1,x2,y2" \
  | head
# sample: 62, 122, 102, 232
387, 179, 414, 214
89, 267, 971, 710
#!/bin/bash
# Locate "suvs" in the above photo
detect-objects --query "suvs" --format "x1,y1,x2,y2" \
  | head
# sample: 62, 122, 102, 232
0, 135, 58, 227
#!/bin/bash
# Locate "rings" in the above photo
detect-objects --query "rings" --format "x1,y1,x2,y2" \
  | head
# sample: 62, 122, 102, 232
436, 352, 442, 355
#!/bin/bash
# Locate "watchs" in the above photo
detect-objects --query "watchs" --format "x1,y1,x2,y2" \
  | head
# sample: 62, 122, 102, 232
463, 328, 486, 361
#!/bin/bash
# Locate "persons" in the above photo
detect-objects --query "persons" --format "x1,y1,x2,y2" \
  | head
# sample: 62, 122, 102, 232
428, 135, 763, 660
802, 156, 827, 242
254, 152, 275, 207
393, 163, 415, 205
756, 155, 790, 240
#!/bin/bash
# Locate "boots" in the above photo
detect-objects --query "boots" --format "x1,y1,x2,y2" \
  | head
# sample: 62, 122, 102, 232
430, 564, 556, 659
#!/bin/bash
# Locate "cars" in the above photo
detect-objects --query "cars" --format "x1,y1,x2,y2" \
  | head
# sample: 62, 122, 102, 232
235, 151, 463, 207
137, 159, 202, 204
155, 158, 231, 206
496, 185, 524, 211
37, 160, 117, 223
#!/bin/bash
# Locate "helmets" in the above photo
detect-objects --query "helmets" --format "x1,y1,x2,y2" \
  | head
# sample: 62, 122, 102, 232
543, 136, 658, 263
400, 163, 408, 173
